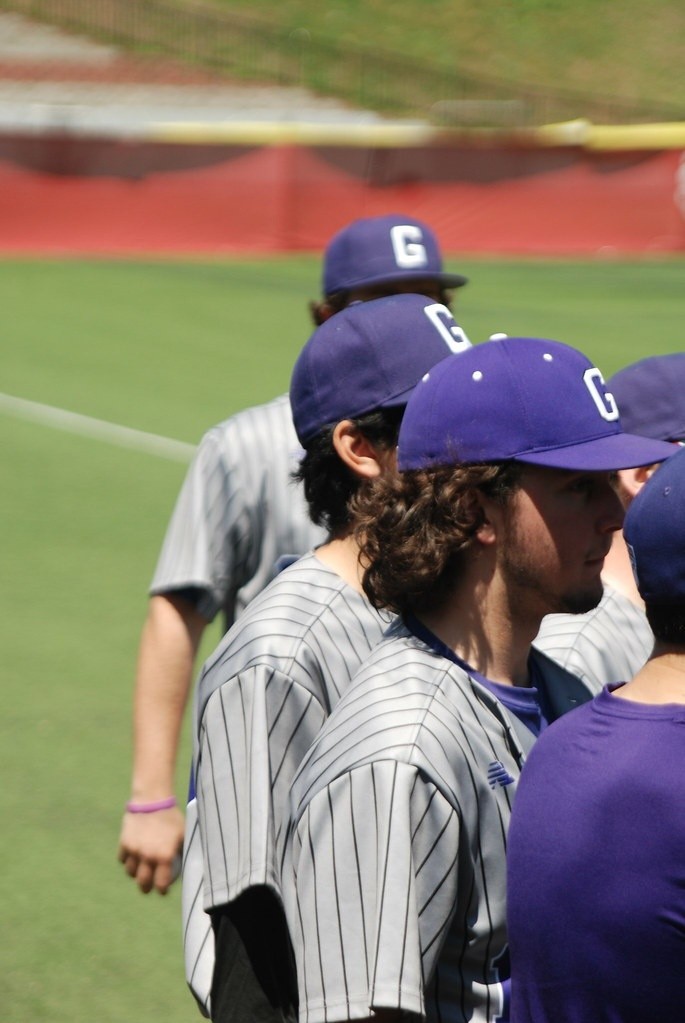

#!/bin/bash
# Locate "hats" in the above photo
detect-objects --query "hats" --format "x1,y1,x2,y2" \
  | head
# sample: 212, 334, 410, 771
606, 352, 685, 440
396, 333, 679, 470
319, 214, 469, 299
621, 445, 685, 599
289, 292, 473, 450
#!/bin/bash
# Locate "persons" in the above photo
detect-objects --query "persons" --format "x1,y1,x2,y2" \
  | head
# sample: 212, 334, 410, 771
278, 334, 685, 1022
182, 294, 470, 1023
506, 444, 685, 1023
531, 350, 685, 696
119, 214, 468, 897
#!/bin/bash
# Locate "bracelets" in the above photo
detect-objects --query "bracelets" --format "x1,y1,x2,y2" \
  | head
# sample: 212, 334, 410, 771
128, 796, 175, 813
